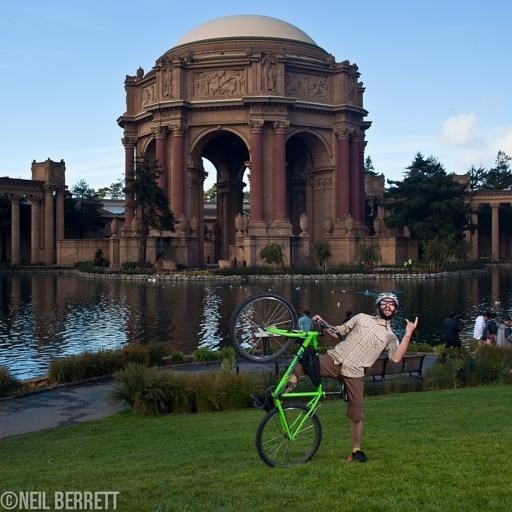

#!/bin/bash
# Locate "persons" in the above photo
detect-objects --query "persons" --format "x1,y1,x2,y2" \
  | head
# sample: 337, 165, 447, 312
340, 310, 352, 325
297, 311, 313, 348
248, 292, 419, 463
440, 309, 512, 362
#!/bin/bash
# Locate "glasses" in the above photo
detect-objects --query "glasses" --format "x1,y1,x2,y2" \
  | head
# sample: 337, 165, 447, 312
379, 301, 395, 306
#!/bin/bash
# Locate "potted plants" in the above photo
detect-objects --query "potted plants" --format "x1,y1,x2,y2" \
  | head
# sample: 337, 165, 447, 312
161, 245, 177, 267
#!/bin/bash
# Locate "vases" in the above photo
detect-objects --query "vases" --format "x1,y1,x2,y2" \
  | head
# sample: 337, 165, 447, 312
217, 259, 232, 270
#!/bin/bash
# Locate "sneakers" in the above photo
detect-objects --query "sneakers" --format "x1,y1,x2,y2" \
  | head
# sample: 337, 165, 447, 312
250, 390, 284, 412
352, 450, 367, 462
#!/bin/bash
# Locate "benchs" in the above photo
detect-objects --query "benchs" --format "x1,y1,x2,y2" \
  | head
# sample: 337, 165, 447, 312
365, 354, 425, 384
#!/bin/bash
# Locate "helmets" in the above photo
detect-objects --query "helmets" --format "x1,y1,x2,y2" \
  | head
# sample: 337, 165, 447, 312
375, 292, 399, 307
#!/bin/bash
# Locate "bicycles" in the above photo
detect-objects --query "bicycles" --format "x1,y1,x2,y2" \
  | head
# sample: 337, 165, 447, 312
228, 294, 350, 468
